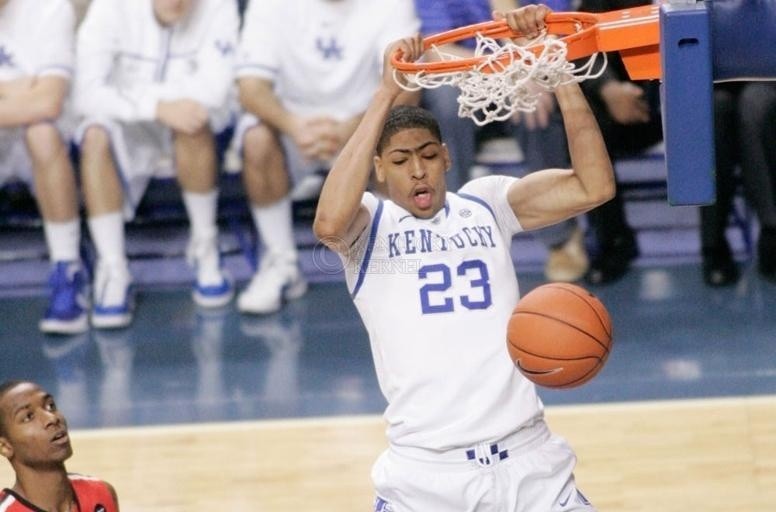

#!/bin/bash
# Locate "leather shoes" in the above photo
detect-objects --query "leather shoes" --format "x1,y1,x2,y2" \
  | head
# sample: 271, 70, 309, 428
704, 232, 738, 285
759, 226, 776, 280
598, 226, 641, 283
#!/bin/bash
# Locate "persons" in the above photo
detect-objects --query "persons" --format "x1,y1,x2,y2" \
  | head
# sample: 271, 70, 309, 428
313, 5, 617, 512
741, 82, 775, 281
42, 327, 90, 428
76, 0, 245, 329
0, 0, 95, 338
572, 0, 738, 289
1, 382, 120, 511
92, 300, 236, 426
239, 311, 308, 420
421, 1, 589, 286
230, 0, 420, 317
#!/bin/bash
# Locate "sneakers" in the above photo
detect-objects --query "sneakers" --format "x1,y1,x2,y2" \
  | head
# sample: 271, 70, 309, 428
237, 246, 308, 315
187, 236, 232, 307
545, 226, 590, 282
38, 262, 88, 333
88, 254, 137, 330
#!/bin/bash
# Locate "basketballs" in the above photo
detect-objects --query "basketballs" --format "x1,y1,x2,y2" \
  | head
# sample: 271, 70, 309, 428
507, 284, 612, 388
